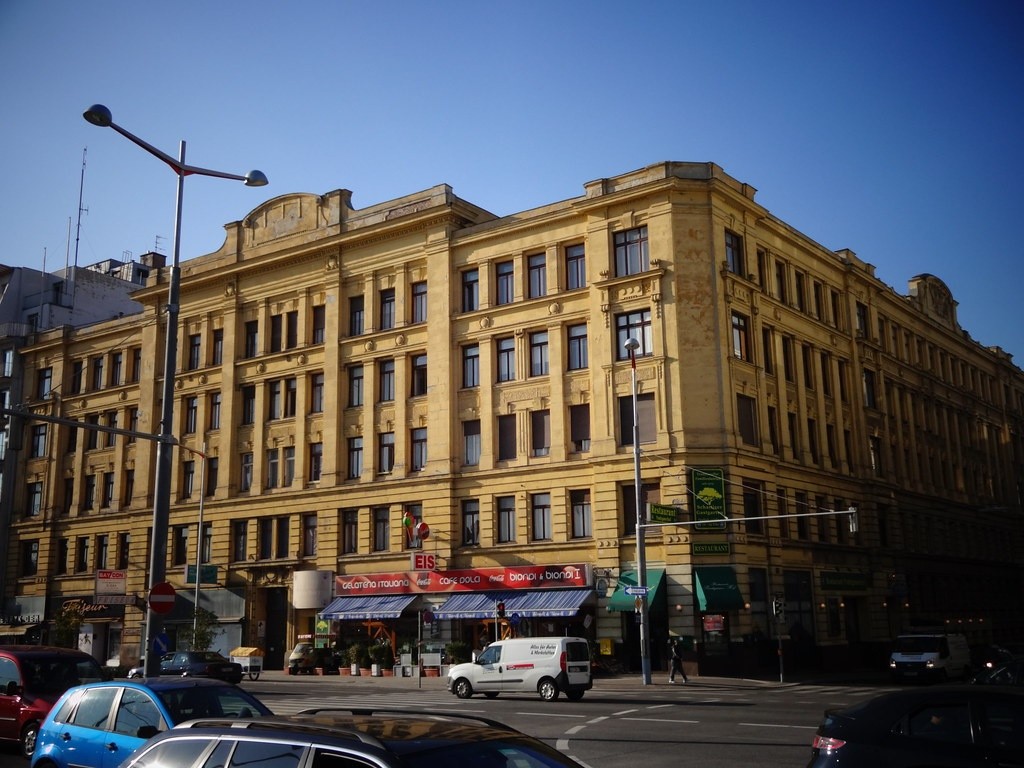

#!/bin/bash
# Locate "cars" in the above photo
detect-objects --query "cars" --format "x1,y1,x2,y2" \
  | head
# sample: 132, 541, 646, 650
126, 651, 242, 684
804, 684, 1024, 768
30, 678, 274, 768
0, 646, 108, 758
82, 104, 270, 678
923, 643, 1020, 685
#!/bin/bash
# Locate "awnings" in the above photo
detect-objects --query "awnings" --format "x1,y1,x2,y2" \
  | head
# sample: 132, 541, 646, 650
433, 589, 593, 620
319, 595, 417, 618
607, 568, 667, 614
693, 567, 744, 612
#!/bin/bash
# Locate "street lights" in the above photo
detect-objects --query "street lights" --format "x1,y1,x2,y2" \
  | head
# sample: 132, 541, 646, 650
623, 337, 652, 685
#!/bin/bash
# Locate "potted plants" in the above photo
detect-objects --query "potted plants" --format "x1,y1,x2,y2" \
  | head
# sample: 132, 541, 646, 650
346, 643, 385, 677
445, 641, 469, 665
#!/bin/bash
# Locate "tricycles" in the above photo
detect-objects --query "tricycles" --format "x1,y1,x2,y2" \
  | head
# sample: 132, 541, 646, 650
288, 642, 330, 675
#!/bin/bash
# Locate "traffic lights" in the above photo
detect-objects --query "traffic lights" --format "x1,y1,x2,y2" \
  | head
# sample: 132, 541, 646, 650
497, 603, 506, 617
774, 599, 783, 618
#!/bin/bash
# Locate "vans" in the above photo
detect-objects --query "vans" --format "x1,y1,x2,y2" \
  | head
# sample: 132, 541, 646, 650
889, 632, 967, 681
446, 636, 595, 701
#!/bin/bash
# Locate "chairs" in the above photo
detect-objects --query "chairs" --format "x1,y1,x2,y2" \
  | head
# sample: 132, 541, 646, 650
125, 691, 210, 734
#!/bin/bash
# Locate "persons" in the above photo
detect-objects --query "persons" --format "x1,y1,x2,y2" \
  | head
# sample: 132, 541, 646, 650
669, 640, 688, 682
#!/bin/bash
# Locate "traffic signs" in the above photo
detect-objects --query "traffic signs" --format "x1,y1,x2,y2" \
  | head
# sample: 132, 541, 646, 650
624, 587, 648, 596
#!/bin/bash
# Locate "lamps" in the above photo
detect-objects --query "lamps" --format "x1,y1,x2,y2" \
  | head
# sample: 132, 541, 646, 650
606, 606, 611, 614
676, 605, 682, 612
744, 603, 751, 611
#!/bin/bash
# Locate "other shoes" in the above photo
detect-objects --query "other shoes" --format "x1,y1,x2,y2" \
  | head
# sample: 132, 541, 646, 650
683, 679, 691, 683
669, 680, 675, 683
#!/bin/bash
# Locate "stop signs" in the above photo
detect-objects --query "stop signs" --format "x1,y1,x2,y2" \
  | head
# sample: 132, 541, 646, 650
149, 583, 176, 615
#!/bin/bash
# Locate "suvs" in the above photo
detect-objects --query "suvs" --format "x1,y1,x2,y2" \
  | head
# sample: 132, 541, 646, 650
117, 707, 586, 768
